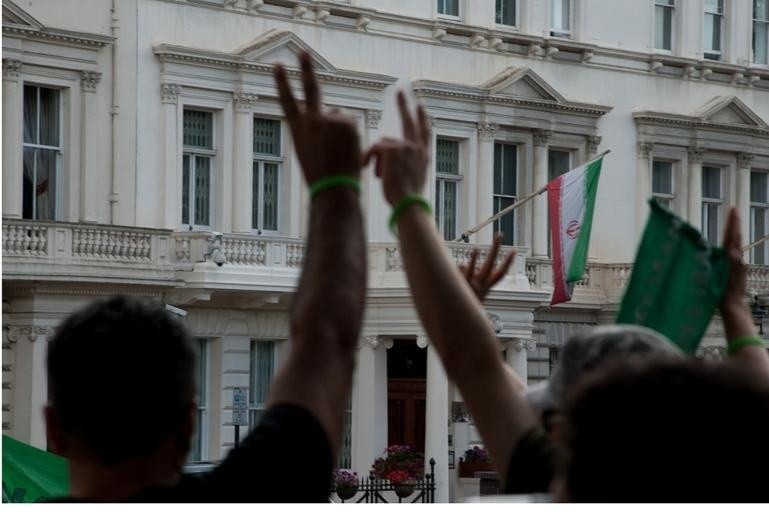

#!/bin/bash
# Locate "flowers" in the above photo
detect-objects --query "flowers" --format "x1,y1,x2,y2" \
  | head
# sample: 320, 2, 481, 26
371, 444, 425, 481
332, 470, 359, 486
464, 444, 491, 463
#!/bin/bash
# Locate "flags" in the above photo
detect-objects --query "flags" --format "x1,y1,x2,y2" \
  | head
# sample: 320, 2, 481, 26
546, 157, 602, 306
3, 433, 71, 503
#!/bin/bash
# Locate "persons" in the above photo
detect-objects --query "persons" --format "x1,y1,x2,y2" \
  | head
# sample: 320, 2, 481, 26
460, 209, 769, 432
33, 47, 363, 504
365, 89, 769, 503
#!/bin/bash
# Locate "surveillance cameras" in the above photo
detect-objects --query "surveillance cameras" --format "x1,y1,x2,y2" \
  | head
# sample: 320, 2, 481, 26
165, 303, 188, 317
213, 251, 226, 266
492, 320, 503, 334
211, 231, 224, 241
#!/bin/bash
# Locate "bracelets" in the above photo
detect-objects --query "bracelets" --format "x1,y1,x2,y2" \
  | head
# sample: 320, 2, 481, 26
308, 177, 357, 193
387, 193, 429, 235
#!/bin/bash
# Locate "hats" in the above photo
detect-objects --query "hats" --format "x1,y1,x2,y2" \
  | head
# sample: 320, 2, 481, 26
519, 323, 689, 416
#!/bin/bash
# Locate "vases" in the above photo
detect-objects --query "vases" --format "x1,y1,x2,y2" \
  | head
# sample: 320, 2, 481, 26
394, 484, 414, 498
336, 488, 357, 499
467, 461, 493, 471
